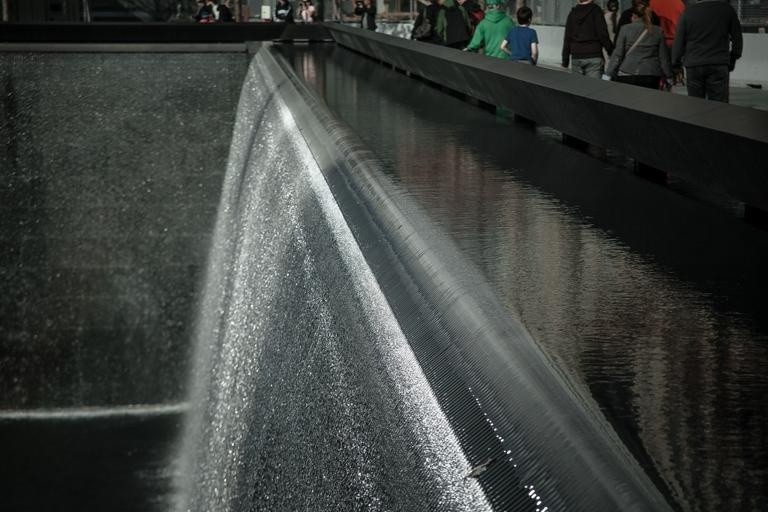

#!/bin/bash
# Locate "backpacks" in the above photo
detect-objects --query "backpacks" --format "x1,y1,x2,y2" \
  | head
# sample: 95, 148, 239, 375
440, 3, 470, 45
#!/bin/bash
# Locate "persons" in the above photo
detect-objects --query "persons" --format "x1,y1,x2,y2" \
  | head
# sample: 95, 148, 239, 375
355, 0, 378, 31
671, 0, 742, 102
192, 0, 325, 23
412, 0, 685, 92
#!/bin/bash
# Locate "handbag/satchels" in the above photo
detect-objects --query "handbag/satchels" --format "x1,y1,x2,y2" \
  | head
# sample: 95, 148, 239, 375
412, 19, 432, 37
